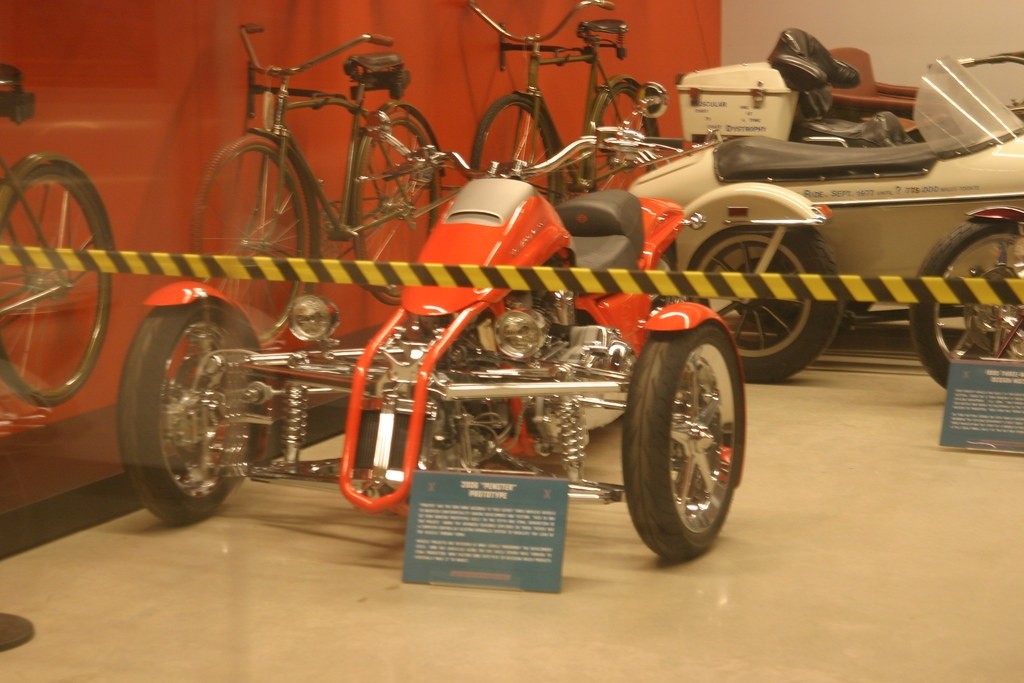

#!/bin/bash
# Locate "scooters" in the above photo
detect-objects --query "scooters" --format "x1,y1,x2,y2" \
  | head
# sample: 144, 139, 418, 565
630, 28, 1022, 366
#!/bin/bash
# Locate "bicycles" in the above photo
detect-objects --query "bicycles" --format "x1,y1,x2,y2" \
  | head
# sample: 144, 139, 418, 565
466, 0, 667, 209
912, 203, 1019, 399
184, 23, 451, 345
0, 68, 111, 416
